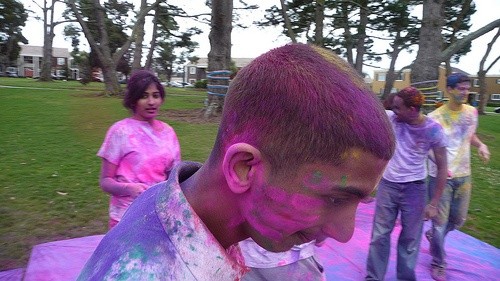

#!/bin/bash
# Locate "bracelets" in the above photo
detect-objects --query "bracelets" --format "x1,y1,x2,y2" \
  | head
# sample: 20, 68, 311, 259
429, 204, 438, 209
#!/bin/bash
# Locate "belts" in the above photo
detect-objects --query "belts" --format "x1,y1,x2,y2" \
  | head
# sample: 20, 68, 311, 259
400, 180, 425, 184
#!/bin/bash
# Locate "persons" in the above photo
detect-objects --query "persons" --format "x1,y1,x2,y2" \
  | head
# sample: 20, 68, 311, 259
426, 73, 490, 281
76, 42, 396, 281
365, 88, 447, 281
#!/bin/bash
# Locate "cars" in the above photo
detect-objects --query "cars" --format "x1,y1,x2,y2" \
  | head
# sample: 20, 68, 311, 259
157, 79, 193, 87
6, 66, 20, 78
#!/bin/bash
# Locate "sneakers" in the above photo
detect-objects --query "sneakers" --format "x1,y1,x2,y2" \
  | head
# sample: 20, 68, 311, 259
431, 264, 447, 281
425, 229, 432, 244
